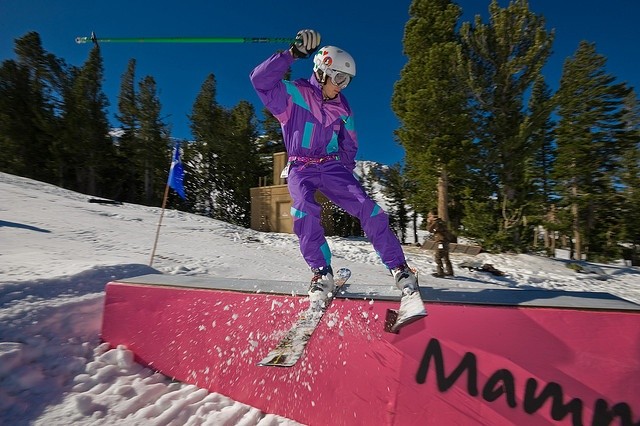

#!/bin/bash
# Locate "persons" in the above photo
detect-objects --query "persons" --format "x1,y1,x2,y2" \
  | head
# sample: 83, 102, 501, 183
250, 28, 417, 293
426, 211, 453, 278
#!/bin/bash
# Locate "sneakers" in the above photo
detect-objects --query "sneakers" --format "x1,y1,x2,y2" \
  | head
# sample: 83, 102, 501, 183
308, 266, 334, 300
390, 262, 418, 295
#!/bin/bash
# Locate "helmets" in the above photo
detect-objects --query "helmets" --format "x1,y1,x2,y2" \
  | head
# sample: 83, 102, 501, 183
313, 46, 356, 84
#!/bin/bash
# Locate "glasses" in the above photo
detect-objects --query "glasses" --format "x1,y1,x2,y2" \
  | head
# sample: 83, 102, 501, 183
315, 60, 351, 87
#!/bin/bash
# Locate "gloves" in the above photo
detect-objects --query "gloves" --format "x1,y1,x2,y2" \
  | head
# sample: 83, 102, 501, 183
292, 29, 321, 59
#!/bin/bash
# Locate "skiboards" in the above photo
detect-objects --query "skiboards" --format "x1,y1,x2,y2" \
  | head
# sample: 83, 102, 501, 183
256, 268, 428, 367
